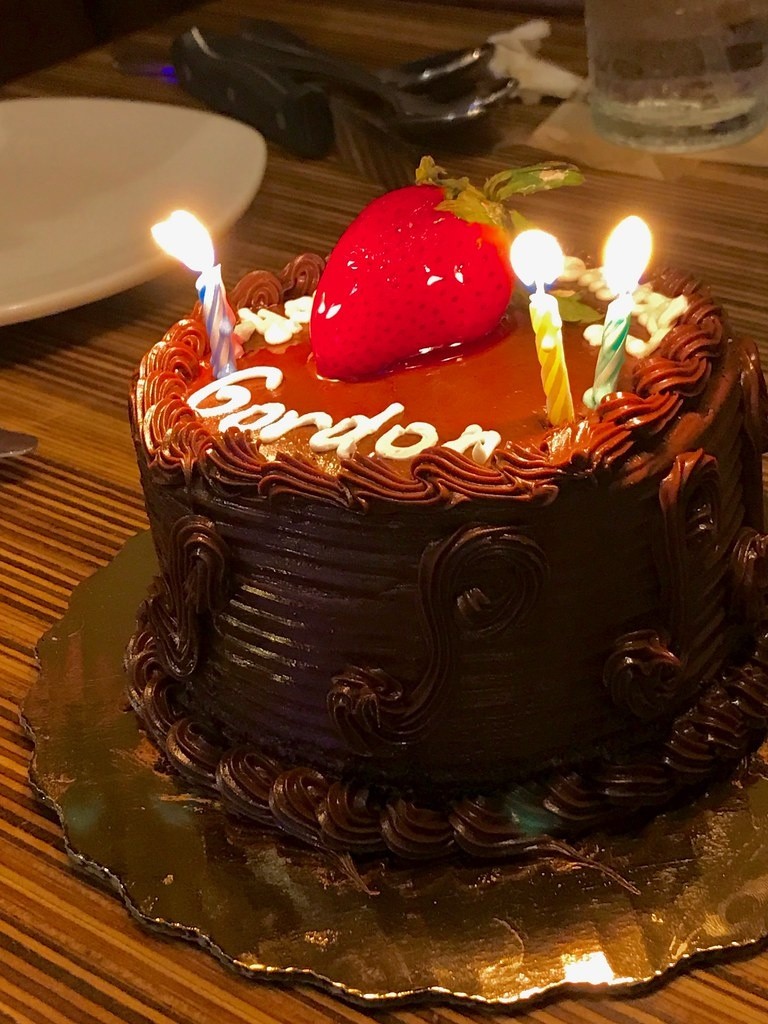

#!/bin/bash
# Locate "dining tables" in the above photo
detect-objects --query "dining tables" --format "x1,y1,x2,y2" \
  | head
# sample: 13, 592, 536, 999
0, 0, 768, 1024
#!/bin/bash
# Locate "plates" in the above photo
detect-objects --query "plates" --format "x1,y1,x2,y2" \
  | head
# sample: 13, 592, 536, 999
0, 98, 268, 326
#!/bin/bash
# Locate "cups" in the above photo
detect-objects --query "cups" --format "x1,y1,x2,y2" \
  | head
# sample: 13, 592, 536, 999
584, 0, 768, 154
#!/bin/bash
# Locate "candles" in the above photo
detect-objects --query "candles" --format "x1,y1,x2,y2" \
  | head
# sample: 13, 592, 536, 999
508, 228, 577, 429
148, 209, 244, 381
580, 215, 654, 409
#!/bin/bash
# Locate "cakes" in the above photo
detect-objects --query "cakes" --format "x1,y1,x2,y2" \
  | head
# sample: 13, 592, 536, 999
112, 156, 768, 863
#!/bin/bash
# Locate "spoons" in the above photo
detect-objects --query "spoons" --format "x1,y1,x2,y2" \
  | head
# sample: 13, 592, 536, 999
188, 25, 516, 134
